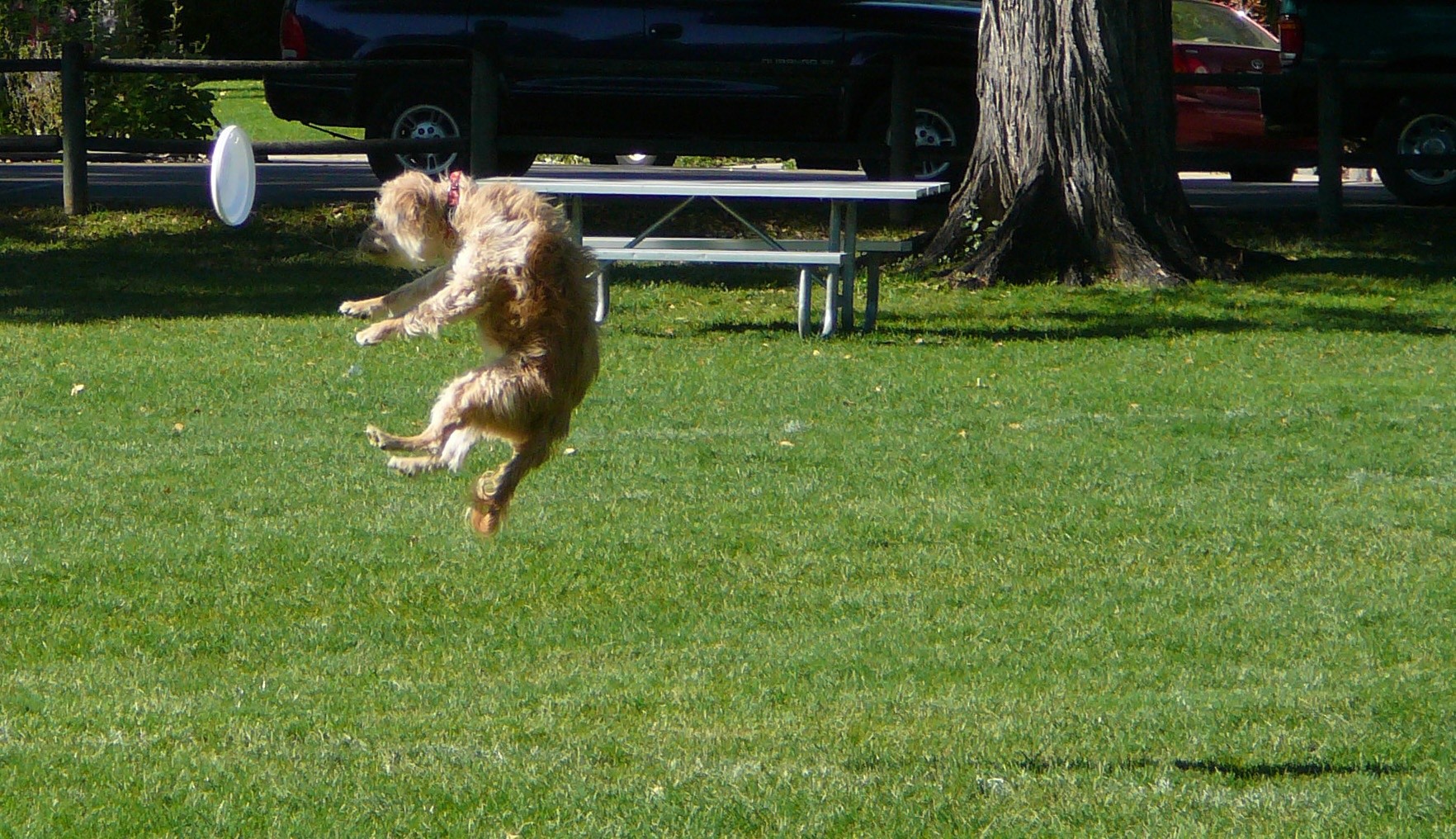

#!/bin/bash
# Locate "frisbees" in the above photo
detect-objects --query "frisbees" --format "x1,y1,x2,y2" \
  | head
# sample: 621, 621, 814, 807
208, 122, 257, 226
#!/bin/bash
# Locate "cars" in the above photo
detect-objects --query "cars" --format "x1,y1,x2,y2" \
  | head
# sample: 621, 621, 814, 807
1170, 0, 1318, 183
261, 0, 983, 182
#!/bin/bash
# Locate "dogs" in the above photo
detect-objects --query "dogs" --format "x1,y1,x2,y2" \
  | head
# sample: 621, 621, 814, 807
336, 167, 601, 540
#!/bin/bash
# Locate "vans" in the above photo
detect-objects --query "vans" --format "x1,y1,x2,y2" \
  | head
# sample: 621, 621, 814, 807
1279, 0, 1456, 204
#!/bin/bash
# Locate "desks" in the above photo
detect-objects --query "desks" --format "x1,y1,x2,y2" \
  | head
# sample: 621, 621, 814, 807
475, 176, 951, 341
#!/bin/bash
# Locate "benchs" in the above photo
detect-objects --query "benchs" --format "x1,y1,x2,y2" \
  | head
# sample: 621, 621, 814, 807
581, 237, 913, 265
